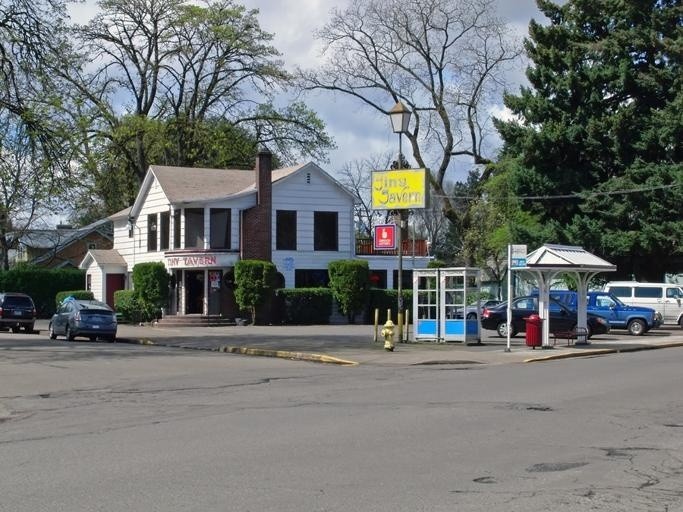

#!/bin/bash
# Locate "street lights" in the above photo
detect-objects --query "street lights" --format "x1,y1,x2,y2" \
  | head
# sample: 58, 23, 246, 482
388, 102, 411, 340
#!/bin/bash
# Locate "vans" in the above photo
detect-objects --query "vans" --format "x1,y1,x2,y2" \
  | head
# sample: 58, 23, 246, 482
603, 283, 683, 328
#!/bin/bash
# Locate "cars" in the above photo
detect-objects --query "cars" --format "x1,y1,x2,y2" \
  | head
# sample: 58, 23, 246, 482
446, 291, 664, 339
0, 292, 36, 331
48, 299, 117, 342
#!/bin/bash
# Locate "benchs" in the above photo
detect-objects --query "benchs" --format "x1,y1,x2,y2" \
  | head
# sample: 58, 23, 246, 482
553, 326, 588, 347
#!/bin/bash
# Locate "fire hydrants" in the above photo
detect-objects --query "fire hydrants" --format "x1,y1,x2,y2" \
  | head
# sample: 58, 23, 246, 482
379, 320, 396, 351
